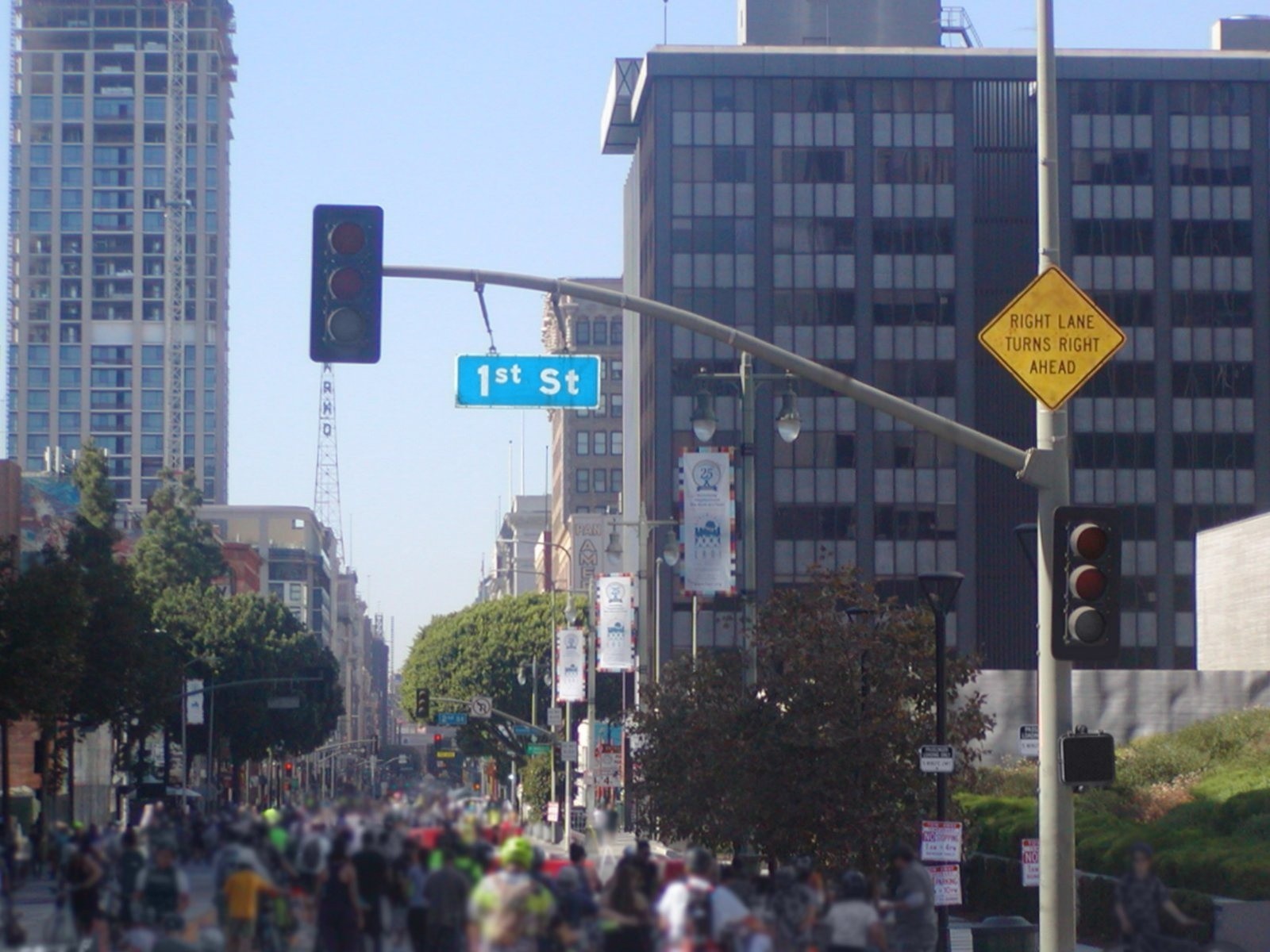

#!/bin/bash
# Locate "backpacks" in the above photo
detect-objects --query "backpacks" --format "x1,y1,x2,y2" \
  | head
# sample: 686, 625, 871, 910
679, 878, 718, 943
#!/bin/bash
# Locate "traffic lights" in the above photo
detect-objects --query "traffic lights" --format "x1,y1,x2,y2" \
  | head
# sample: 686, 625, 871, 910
1059, 730, 1116, 787
1049, 503, 1124, 665
307, 201, 385, 369
416, 687, 429, 718
285, 762, 293, 778
434, 733, 441, 750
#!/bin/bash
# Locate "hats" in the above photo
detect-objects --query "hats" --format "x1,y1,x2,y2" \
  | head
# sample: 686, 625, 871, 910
500, 837, 533, 865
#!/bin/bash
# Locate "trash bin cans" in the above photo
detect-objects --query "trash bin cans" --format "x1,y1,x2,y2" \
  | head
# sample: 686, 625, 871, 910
969, 915, 1040, 952
595, 809, 618, 834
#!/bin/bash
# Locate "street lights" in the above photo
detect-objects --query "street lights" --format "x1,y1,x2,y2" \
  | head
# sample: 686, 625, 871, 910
688, 348, 806, 877
514, 653, 553, 745
916, 569, 968, 951
495, 535, 574, 856
491, 567, 558, 845
845, 602, 889, 901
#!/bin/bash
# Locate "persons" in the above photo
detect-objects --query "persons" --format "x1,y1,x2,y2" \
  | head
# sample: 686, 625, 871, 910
0, 797, 941, 952
1116, 845, 1201, 952
